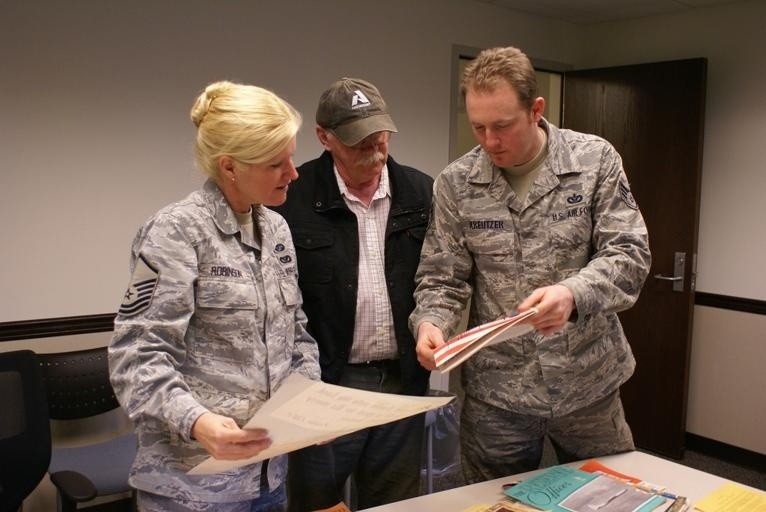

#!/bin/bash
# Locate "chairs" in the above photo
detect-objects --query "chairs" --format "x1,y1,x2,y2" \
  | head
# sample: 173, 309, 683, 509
1, 345, 139, 511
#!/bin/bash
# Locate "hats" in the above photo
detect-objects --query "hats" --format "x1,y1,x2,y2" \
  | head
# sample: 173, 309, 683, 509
315, 76, 398, 147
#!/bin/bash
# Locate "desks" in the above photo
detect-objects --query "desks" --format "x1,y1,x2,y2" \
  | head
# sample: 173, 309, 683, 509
356, 451, 765, 512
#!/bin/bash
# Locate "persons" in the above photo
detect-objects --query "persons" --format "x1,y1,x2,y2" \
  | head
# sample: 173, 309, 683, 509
263, 76, 434, 512
407, 47, 652, 485
107, 81, 326, 512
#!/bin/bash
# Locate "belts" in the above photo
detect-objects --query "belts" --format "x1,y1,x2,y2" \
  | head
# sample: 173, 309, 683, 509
359, 358, 391, 368
